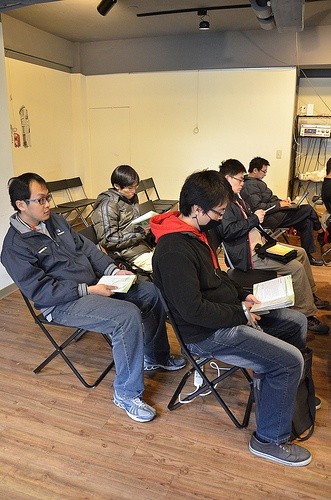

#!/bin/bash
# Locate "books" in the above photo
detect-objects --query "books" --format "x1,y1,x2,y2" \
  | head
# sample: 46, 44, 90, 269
266, 242, 299, 256
96, 274, 138, 293
132, 211, 160, 231
249, 274, 296, 314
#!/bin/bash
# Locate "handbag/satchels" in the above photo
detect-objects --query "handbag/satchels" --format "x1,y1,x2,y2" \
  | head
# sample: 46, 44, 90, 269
253, 347, 316, 442
258, 241, 296, 262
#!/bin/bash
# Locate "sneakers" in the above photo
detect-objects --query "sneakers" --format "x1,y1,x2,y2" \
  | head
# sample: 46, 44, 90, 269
113, 389, 156, 422
248, 432, 312, 467
307, 316, 329, 333
314, 296, 329, 308
315, 397, 321, 409
143, 354, 187, 370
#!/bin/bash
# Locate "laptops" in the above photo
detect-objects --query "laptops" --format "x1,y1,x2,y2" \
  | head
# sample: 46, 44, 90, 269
290, 191, 307, 209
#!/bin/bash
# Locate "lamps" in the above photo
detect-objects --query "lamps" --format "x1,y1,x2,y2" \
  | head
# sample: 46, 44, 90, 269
197, 10, 210, 30
96, 0, 117, 16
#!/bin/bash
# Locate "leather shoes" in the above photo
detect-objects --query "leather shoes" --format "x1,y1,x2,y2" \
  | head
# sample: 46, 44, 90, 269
310, 257, 323, 265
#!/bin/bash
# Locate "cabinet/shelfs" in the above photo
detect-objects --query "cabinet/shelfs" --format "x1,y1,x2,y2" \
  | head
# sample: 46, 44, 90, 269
288, 115, 331, 200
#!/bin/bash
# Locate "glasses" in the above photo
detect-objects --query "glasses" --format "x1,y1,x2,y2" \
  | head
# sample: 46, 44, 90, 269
119, 185, 140, 191
24, 193, 52, 205
230, 176, 245, 182
259, 168, 267, 174
210, 209, 226, 218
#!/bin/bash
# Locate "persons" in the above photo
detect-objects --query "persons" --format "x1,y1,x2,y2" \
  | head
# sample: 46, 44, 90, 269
91, 164, 175, 326
240, 156, 331, 266
239, 158, 331, 266
219, 159, 330, 334
146, 169, 322, 467
1, 172, 188, 422
321, 158, 331, 214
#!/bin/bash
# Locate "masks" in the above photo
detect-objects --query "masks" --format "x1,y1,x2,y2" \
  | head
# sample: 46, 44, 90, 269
196, 207, 223, 232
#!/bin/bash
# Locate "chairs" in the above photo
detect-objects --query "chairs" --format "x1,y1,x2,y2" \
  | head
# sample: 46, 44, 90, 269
19, 177, 290, 429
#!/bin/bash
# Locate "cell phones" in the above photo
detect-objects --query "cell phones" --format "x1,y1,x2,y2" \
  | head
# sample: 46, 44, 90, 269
264, 205, 276, 212
